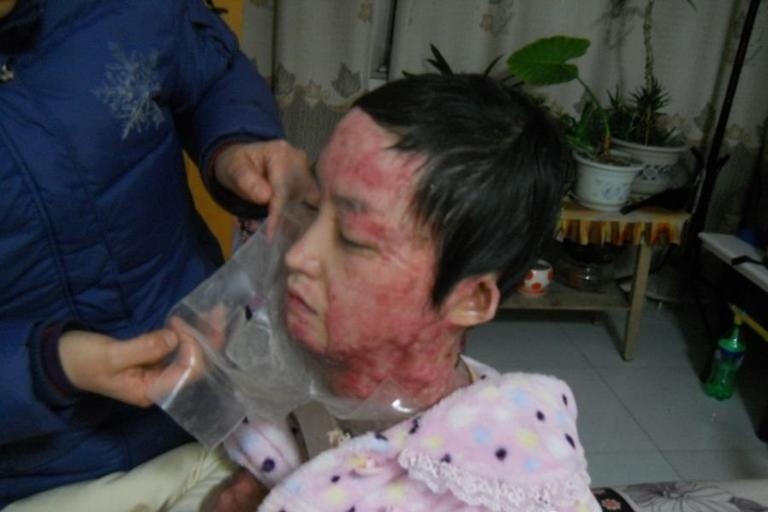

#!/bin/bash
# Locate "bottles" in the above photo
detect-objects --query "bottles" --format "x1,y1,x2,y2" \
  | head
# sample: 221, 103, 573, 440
704, 314, 748, 400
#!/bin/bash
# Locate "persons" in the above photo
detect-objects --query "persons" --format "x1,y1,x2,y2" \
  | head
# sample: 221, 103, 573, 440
1, 0, 312, 509
199, 73, 604, 510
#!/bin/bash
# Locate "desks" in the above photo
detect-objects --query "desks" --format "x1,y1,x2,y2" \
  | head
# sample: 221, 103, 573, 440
500, 200, 686, 362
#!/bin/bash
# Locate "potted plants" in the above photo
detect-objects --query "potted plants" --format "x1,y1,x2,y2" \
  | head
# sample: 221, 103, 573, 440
507, 35, 646, 216
601, 3, 692, 194
